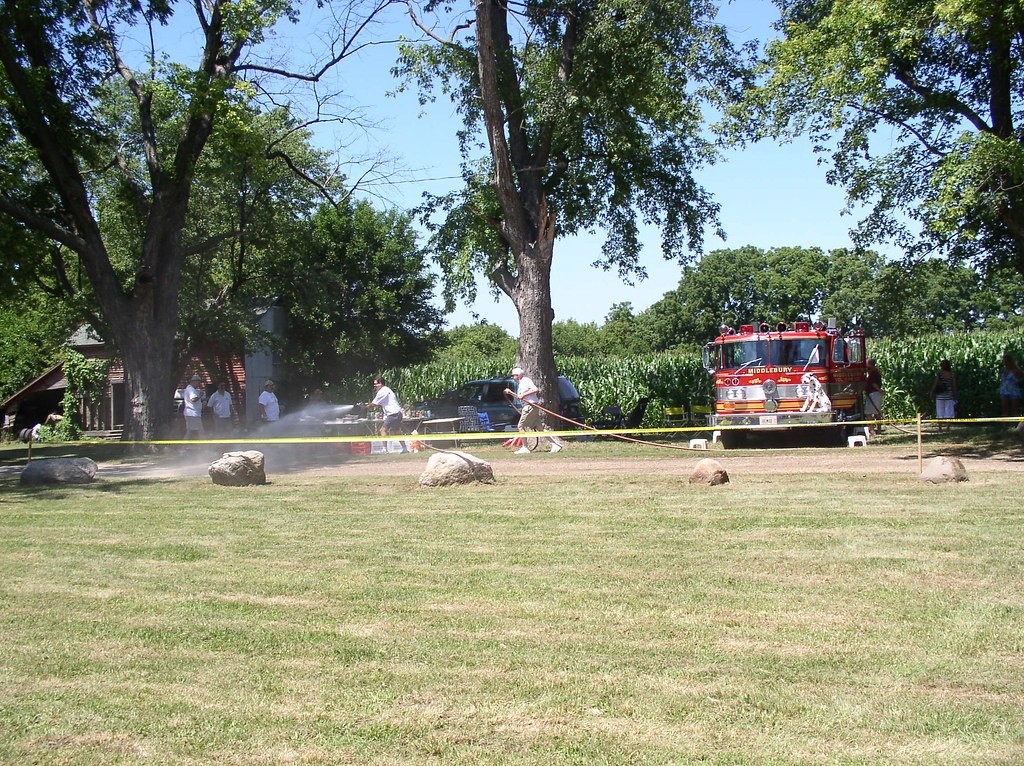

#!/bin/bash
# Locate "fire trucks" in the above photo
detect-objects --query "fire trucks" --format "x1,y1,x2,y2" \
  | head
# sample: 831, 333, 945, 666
702, 319, 868, 446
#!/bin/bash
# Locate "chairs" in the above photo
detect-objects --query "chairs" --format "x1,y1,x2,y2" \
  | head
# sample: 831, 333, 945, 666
663, 405, 690, 439
458, 405, 498, 446
592, 398, 649, 439
690, 406, 713, 439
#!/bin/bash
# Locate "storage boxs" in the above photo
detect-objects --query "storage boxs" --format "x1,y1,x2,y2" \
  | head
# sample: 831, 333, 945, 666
351, 439, 418, 455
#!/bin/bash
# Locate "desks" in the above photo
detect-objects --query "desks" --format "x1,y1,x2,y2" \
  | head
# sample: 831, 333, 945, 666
321, 417, 466, 450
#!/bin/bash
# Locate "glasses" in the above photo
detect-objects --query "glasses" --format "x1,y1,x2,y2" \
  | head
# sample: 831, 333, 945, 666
511, 374, 514, 377
221, 384, 226, 386
374, 383, 379, 385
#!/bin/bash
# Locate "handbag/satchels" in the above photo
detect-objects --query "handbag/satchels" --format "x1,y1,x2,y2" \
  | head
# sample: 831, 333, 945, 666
935, 384, 945, 393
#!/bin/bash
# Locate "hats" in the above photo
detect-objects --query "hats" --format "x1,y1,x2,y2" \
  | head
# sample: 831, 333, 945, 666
191, 375, 204, 382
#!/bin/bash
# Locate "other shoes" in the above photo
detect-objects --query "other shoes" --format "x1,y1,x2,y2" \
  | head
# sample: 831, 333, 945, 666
514, 446, 530, 455
400, 451, 409, 454
550, 442, 563, 453
378, 450, 389, 455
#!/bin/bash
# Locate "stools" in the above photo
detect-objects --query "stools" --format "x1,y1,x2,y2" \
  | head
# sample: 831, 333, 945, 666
713, 431, 722, 443
854, 427, 870, 440
848, 435, 866, 447
690, 439, 709, 451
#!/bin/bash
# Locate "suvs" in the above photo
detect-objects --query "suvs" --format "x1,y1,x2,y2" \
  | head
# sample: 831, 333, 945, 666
413, 377, 582, 429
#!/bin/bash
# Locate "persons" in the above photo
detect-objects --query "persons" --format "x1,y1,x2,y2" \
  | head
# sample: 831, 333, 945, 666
258, 380, 280, 423
300, 388, 328, 437
361, 376, 409, 454
504, 367, 564, 454
462, 386, 475, 402
997, 355, 1024, 427
179, 373, 207, 453
207, 381, 239, 433
863, 359, 882, 434
929, 360, 958, 433
45, 411, 65, 431
